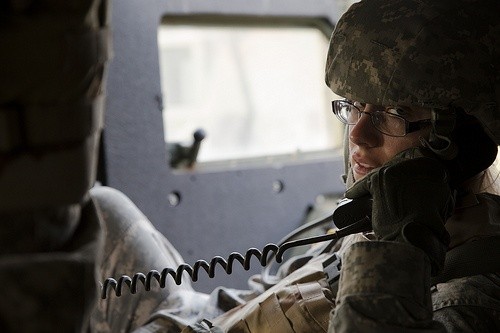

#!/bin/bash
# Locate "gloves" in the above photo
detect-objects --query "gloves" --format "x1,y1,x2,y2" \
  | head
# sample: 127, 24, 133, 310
344, 146, 459, 266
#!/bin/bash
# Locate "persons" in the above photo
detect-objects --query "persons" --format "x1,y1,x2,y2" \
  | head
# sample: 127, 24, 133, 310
83, 0, 500, 333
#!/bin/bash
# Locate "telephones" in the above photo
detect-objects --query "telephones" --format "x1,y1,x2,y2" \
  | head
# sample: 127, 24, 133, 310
332, 118, 497, 234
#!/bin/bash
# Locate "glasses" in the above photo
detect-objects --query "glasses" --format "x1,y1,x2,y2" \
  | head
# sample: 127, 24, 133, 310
332, 100, 433, 137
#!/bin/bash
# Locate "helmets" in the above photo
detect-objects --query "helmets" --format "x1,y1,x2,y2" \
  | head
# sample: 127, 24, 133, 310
326, 0, 500, 141
